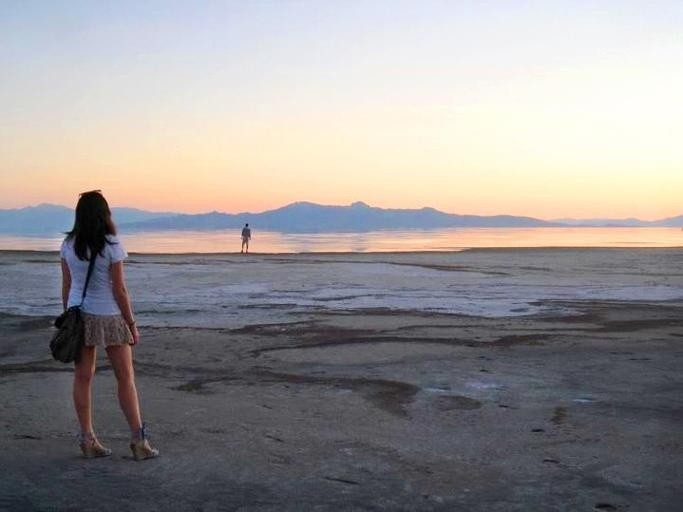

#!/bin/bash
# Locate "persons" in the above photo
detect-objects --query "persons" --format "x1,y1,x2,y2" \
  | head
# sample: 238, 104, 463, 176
56, 189, 159, 460
239, 222, 251, 256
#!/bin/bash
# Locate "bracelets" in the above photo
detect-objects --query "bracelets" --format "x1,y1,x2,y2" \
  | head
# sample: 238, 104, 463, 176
127, 320, 136, 325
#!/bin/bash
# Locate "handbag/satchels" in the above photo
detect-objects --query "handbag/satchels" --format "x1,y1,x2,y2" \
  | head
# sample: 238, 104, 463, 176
48, 305, 84, 363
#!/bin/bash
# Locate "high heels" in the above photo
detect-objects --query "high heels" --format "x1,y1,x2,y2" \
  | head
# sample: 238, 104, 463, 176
129, 427, 159, 460
80, 431, 111, 458
